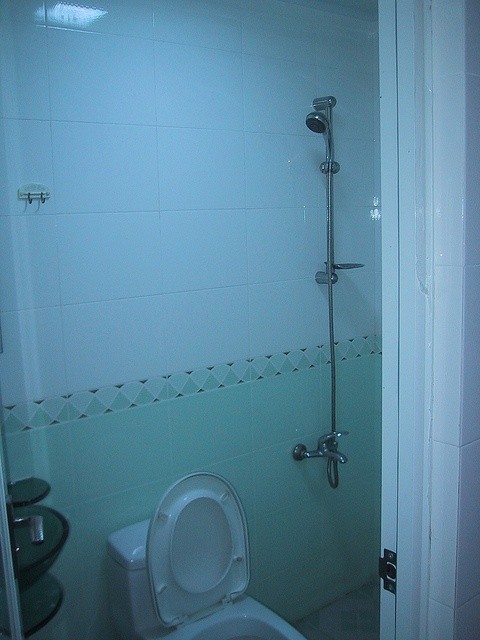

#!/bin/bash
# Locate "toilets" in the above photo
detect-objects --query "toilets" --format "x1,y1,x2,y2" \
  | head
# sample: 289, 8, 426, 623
108, 474, 303, 636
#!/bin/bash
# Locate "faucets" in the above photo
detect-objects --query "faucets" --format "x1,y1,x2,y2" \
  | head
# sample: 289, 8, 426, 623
299, 432, 350, 463
